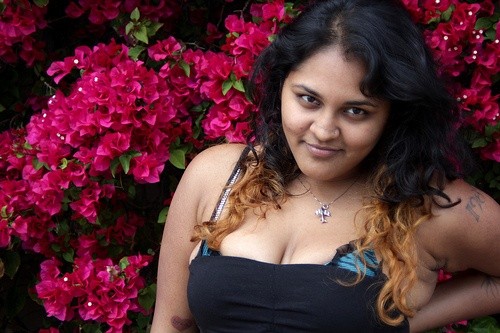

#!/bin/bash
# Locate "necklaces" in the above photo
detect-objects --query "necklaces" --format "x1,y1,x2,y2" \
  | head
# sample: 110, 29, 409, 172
298, 177, 359, 224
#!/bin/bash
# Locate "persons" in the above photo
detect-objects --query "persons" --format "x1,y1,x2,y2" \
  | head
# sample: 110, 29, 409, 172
149, 0, 499, 333
